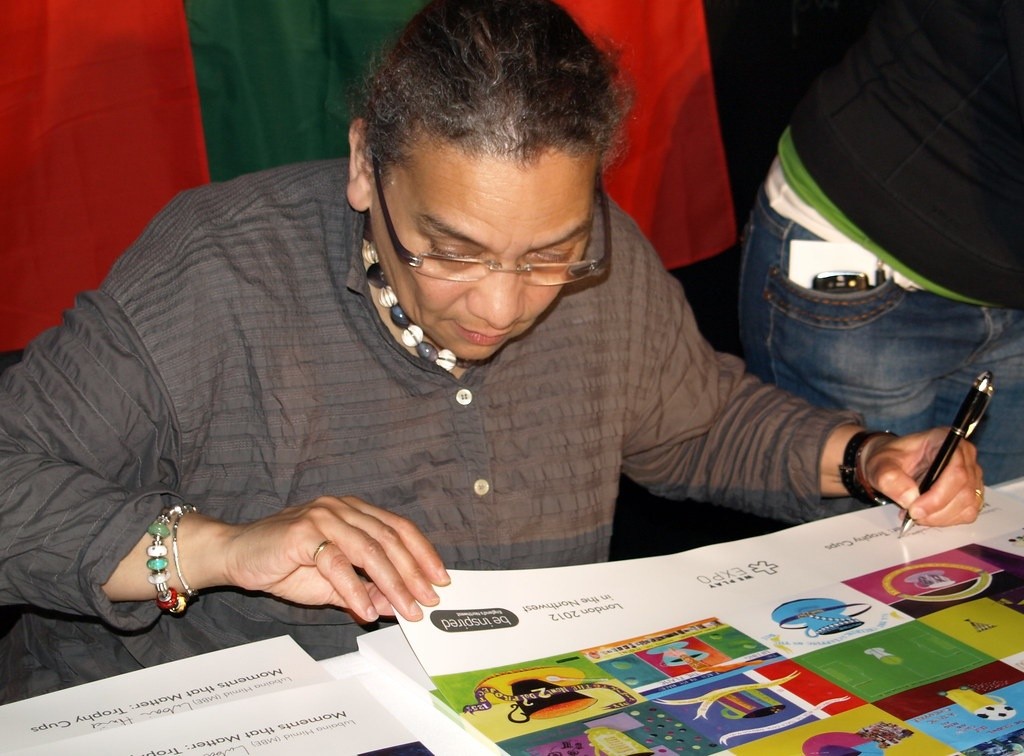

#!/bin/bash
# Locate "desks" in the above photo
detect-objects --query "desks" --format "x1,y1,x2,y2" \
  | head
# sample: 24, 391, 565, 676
0, 476, 1024, 753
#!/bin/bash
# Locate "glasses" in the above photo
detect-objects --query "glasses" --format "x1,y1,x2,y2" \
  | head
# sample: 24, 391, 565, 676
371, 145, 611, 286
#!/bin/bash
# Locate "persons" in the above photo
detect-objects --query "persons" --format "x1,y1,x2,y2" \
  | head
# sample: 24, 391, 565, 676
733, 0, 1024, 488
0, 0, 983, 706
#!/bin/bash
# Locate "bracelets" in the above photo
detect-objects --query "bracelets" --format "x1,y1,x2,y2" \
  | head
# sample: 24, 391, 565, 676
837, 428, 902, 507
147, 503, 200, 617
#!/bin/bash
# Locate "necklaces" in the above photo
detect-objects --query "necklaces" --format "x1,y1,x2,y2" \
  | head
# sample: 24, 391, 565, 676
361, 222, 476, 370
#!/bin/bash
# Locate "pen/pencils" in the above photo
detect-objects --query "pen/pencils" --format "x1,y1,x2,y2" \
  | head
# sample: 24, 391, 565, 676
898, 370, 995, 540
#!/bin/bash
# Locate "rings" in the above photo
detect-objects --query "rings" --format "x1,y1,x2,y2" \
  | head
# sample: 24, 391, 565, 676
312, 540, 333, 564
975, 489, 986, 512
